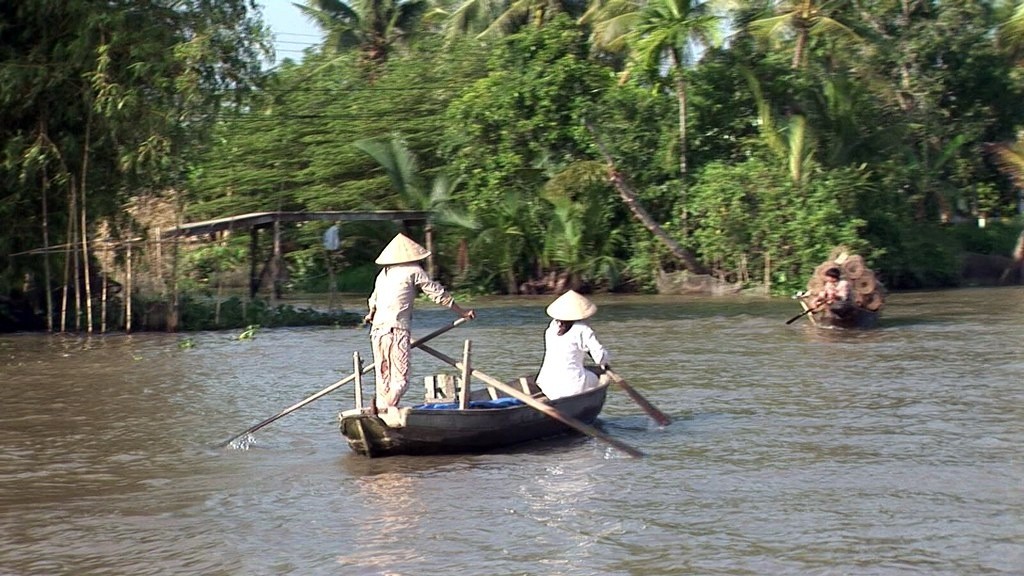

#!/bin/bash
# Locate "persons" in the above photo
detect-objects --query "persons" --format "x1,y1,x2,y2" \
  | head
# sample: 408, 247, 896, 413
812, 269, 853, 306
535, 289, 611, 398
363, 233, 476, 414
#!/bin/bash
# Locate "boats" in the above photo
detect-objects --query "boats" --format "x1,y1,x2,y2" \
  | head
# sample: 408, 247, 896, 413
800, 298, 880, 331
338, 365, 612, 460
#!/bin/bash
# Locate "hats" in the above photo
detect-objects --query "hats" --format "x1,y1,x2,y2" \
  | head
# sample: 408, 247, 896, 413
546, 290, 597, 321
375, 232, 432, 266
